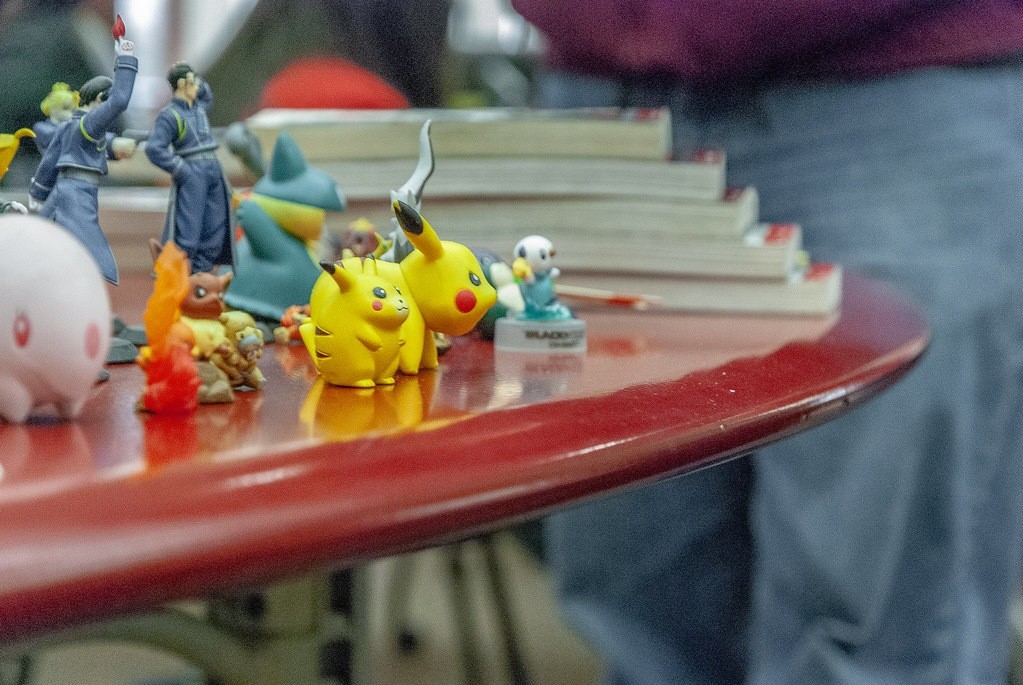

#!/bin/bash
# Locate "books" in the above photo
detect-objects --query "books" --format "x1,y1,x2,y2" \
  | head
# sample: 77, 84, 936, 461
236, 102, 843, 318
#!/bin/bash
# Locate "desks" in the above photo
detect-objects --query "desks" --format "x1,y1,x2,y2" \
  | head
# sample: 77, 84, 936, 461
2, 267, 937, 641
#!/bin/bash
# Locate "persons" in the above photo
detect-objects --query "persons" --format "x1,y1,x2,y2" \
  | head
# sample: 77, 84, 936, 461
25, 14, 138, 283
494, 3, 1021, 685
150, 63, 233, 278
